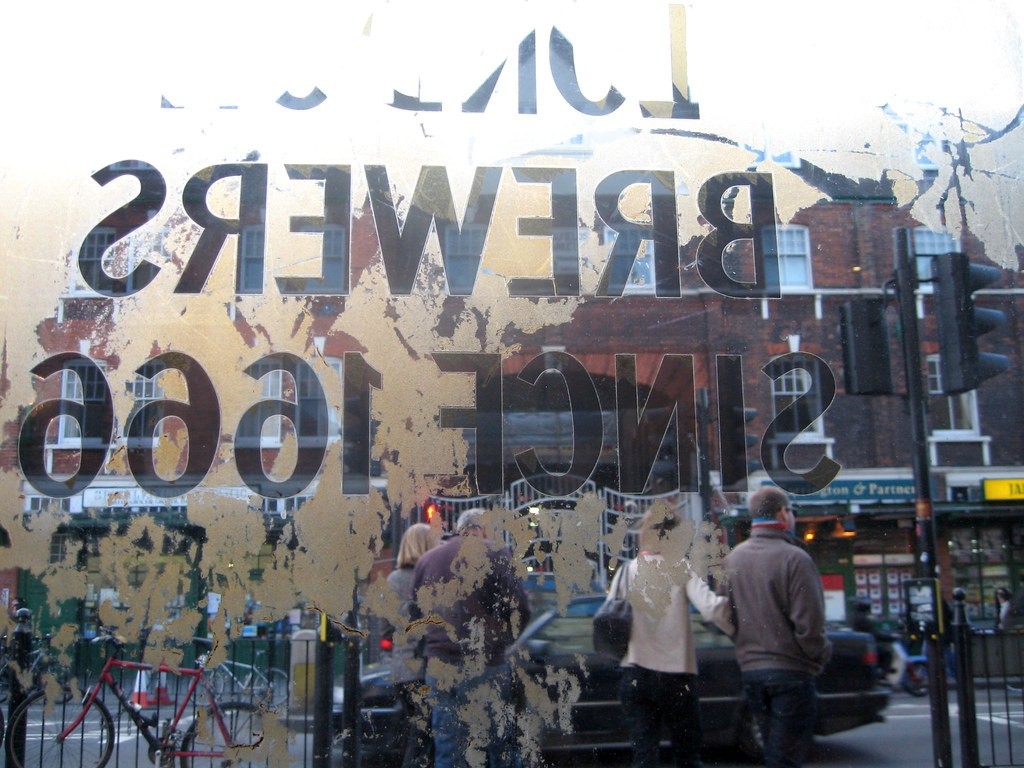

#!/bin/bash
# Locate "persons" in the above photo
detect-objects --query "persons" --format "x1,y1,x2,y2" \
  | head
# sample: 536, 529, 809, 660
407, 508, 532, 768
0, 597, 27, 639
700, 485, 833, 768
850, 595, 905, 680
383, 523, 439, 768
993, 588, 1012, 631
605, 511, 735, 768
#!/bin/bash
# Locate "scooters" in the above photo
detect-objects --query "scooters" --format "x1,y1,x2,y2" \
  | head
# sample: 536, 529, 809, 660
876, 631, 928, 698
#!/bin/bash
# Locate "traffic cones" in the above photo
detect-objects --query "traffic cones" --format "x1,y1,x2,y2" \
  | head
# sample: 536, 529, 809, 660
131, 659, 151, 707
148, 659, 174, 706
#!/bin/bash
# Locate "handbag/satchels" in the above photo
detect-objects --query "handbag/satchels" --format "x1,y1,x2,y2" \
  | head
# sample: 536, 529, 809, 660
592, 561, 633, 658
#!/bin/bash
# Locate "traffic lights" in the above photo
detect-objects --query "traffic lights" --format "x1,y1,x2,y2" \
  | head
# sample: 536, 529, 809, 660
426, 504, 437, 522
933, 254, 1012, 394
380, 638, 392, 650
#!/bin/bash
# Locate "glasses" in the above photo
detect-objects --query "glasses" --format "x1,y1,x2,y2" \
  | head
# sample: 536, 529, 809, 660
778, 507, 798, 518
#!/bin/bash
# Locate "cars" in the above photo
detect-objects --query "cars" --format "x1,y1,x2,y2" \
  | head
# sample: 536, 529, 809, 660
343, 595, 895, 768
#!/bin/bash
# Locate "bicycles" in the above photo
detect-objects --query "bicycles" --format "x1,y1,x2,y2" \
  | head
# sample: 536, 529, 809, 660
5, 635, 273, 768
0, 634, 78, 705
188, 649, 290, 706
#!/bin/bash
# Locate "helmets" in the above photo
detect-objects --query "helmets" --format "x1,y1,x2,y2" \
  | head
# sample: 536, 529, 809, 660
849, 594, 871, 616
